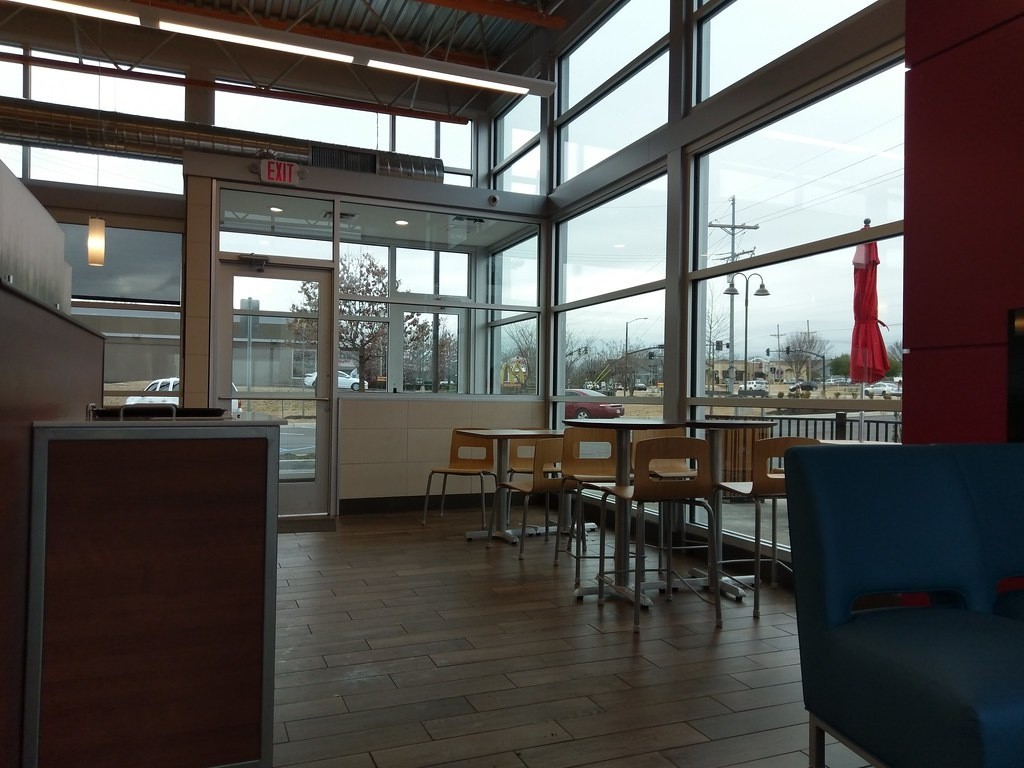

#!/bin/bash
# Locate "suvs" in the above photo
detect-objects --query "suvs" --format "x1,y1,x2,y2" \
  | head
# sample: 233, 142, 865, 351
125, 377, 242, 421
737, 380, 770, 398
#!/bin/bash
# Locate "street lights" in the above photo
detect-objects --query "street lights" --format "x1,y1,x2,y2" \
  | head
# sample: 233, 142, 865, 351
625, 317, 648, 390
722, 272, 770, 398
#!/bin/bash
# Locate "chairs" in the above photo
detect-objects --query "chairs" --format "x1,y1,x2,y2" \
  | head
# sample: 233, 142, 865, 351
422, 426, 1024, 768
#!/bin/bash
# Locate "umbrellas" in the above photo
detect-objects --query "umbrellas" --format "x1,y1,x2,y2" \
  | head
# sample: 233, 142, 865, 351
851, 218, 891, 442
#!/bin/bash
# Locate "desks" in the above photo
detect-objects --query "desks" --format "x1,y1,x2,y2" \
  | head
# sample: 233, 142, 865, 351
456, 430, 597, 546
559, 416, 783, 610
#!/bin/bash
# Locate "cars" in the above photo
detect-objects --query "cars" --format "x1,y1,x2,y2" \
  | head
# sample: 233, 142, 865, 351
789, 381, 819, 392
821, 378, 840, 386
565, 388, 625, 419
305, 368, 370, 391
865, 381, 903, 396
634, 383, 647, 391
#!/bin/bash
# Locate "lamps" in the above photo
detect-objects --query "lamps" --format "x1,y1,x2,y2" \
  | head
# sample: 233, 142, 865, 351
88, 210, 104, 267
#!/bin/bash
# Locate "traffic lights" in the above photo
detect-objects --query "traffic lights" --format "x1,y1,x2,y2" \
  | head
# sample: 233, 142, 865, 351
786, 346, 789, 355
766, 348, 769, 356
